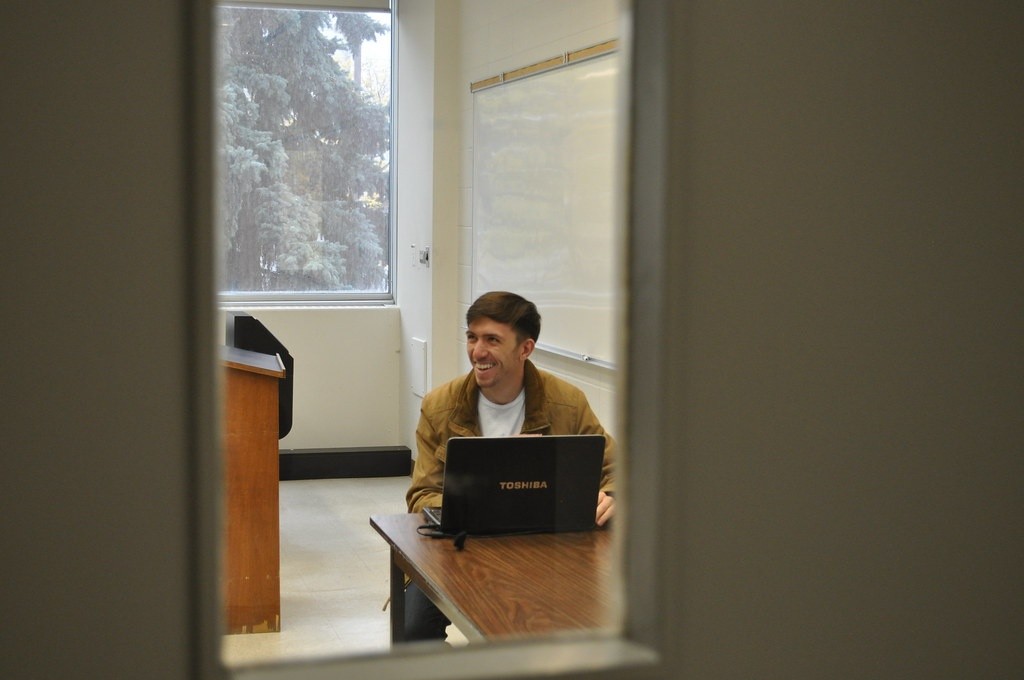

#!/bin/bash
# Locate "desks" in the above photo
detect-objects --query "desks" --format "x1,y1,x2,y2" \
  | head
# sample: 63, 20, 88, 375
370, 514, 614, 649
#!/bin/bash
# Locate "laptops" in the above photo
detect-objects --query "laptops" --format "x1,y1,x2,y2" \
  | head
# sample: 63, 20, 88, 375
422, 433, 606, 536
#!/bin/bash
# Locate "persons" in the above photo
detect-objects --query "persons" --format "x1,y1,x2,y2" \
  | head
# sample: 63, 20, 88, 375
403, 291, 617, 643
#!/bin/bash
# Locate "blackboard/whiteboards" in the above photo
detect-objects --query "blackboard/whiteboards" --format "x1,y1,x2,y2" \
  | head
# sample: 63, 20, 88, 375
468, 34, 623, 375
